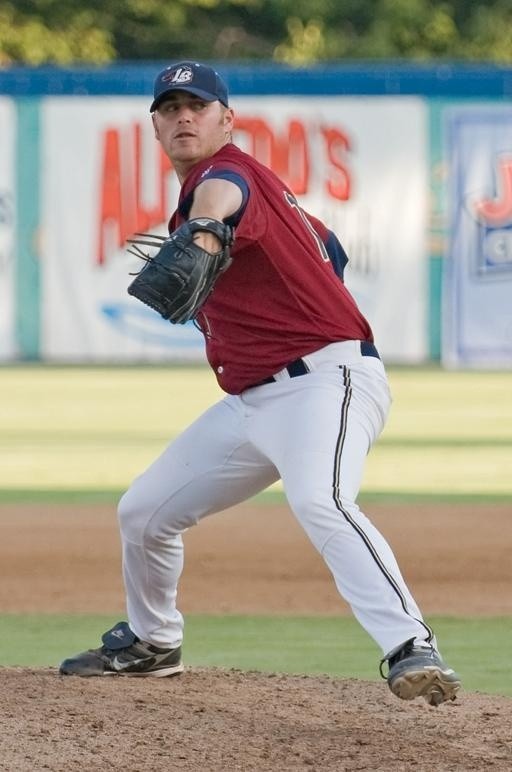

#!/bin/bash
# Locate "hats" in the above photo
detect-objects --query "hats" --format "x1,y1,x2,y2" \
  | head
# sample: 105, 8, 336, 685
149, 62, 228, 112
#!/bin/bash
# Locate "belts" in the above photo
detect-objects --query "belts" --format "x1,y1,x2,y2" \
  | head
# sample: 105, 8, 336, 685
251, 338, 380, 386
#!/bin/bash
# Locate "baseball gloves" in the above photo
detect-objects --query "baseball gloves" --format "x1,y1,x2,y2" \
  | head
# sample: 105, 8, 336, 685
126, 216, 236, 341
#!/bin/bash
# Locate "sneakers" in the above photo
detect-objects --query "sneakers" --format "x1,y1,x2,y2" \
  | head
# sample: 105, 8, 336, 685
385, 649, 461, 705
59, 622, 183, 679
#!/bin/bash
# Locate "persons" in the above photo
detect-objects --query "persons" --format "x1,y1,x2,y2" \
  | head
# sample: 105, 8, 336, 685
57, 60, 464, 708
